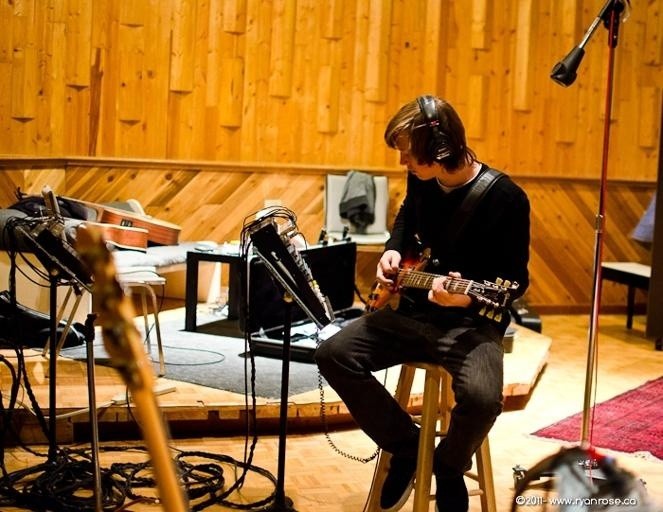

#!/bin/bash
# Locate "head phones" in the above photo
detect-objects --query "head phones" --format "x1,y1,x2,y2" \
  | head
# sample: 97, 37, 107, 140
416, 95, 456, 163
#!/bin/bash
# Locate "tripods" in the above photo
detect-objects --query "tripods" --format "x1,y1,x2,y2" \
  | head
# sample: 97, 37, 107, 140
0, 266, 113, 501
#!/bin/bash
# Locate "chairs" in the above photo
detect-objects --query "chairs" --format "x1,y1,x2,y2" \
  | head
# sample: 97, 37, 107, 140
39, 183, 171, 381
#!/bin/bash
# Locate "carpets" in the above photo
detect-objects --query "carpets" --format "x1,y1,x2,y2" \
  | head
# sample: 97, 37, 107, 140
529, 369, 663, 464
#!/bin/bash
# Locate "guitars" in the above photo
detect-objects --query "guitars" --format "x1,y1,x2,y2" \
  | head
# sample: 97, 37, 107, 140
363, 253, 520, 324
13, 186, 181, 253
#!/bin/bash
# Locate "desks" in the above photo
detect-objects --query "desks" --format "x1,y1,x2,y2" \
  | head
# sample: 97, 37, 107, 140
184, 234, 250, 336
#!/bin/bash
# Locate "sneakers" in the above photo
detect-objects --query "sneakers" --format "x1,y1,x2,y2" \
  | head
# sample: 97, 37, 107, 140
432, 451, 469, 512
380, 453, 416, 512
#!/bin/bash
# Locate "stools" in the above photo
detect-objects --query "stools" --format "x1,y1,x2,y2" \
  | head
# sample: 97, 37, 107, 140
596, 254, 655, 334
362, 345, 502, 512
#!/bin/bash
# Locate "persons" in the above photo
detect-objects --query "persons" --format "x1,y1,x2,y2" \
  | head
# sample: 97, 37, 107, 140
314, 94, 531, 512
